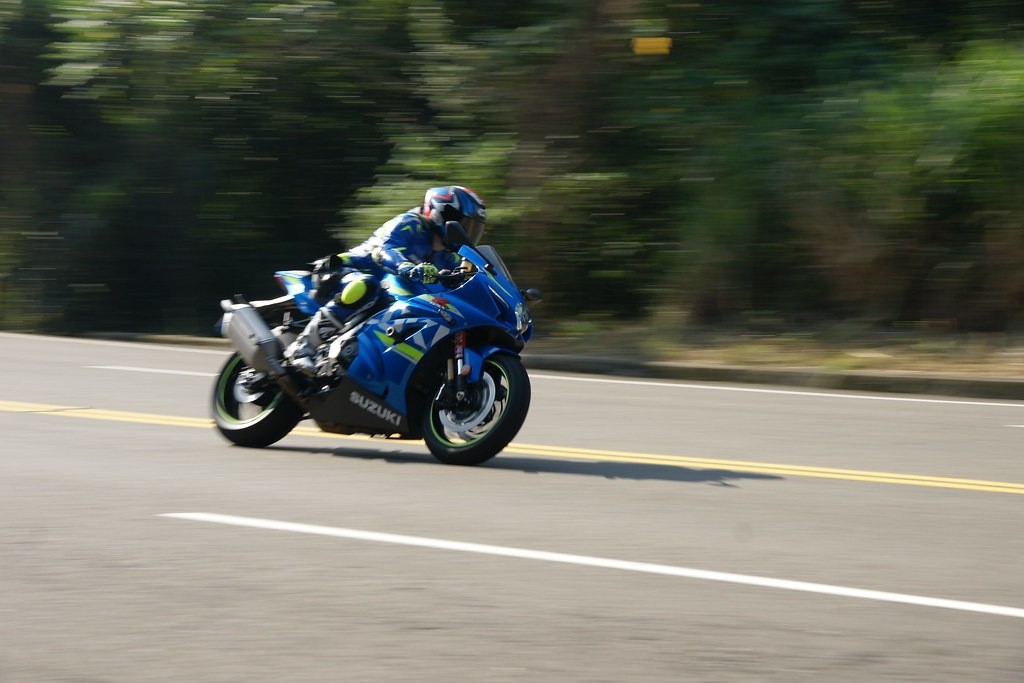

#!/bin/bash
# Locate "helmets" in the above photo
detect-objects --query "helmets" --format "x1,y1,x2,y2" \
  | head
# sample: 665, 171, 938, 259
422, 185, 487, 251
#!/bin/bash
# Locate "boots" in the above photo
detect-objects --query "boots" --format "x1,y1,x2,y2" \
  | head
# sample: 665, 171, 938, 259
283, 306, 345, 377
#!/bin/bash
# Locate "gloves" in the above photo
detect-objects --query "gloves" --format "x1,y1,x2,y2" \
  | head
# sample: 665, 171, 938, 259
411, 262, 438, 284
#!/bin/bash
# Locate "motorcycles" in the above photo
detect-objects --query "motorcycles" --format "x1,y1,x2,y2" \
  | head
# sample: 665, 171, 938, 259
210, 244, 543, 465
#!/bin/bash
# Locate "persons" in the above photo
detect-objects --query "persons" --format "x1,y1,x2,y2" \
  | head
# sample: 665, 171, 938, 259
283, 184, 487, 379
